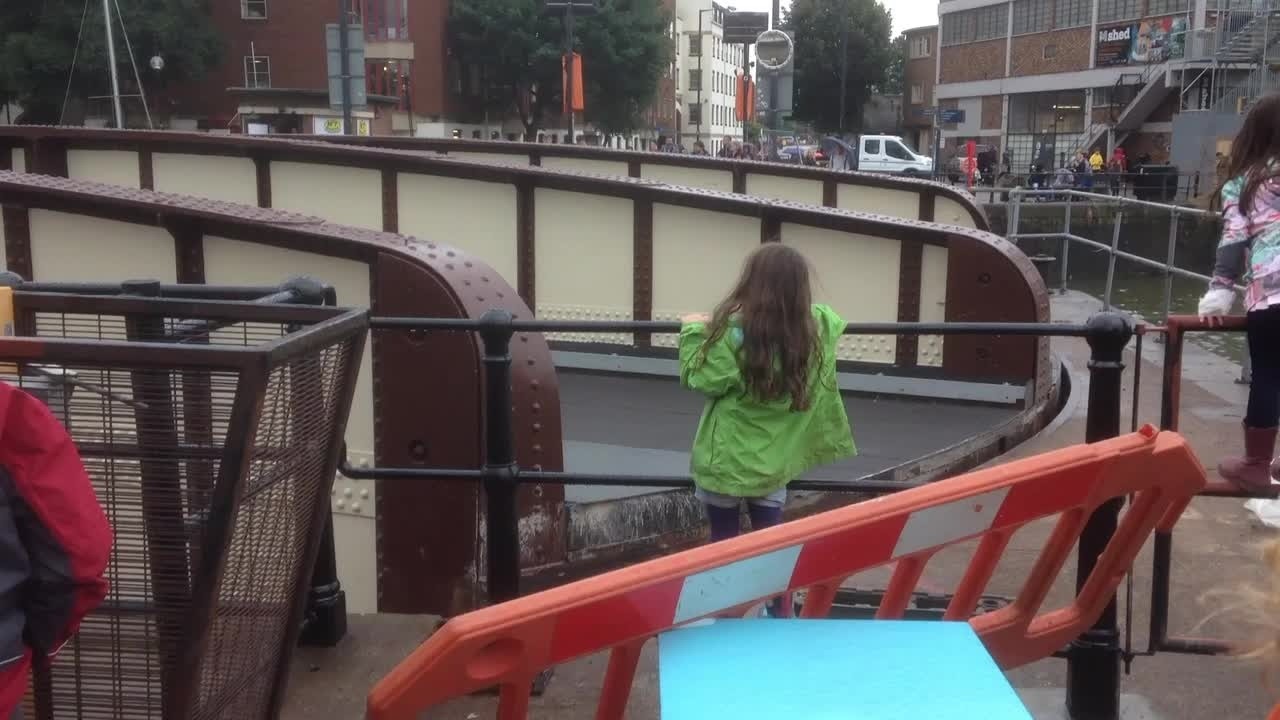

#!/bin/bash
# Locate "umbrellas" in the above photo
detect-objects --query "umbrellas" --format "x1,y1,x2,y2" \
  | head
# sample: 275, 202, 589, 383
821, 136, 857, 170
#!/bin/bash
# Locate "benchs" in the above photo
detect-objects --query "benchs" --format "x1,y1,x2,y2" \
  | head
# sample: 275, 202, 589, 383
1022, 183, 1109, 203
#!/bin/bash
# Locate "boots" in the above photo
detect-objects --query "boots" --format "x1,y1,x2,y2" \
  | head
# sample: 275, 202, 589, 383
1217, 417, 1278, 494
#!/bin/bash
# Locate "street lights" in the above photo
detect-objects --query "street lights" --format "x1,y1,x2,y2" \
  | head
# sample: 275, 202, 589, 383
696, 6, 736, 139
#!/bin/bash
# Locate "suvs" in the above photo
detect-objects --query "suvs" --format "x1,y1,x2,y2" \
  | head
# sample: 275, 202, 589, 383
946, 145, 991, 182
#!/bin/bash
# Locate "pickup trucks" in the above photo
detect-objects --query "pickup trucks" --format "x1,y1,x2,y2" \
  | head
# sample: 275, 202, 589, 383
775, 134, 936, 179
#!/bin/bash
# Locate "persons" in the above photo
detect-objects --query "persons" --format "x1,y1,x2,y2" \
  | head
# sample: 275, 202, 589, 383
678, 241, 858, 618
986, 90, 1230, 212
1199, 91, 1280, 493
0, 381, 114, 720
577, 135, 850, 171
